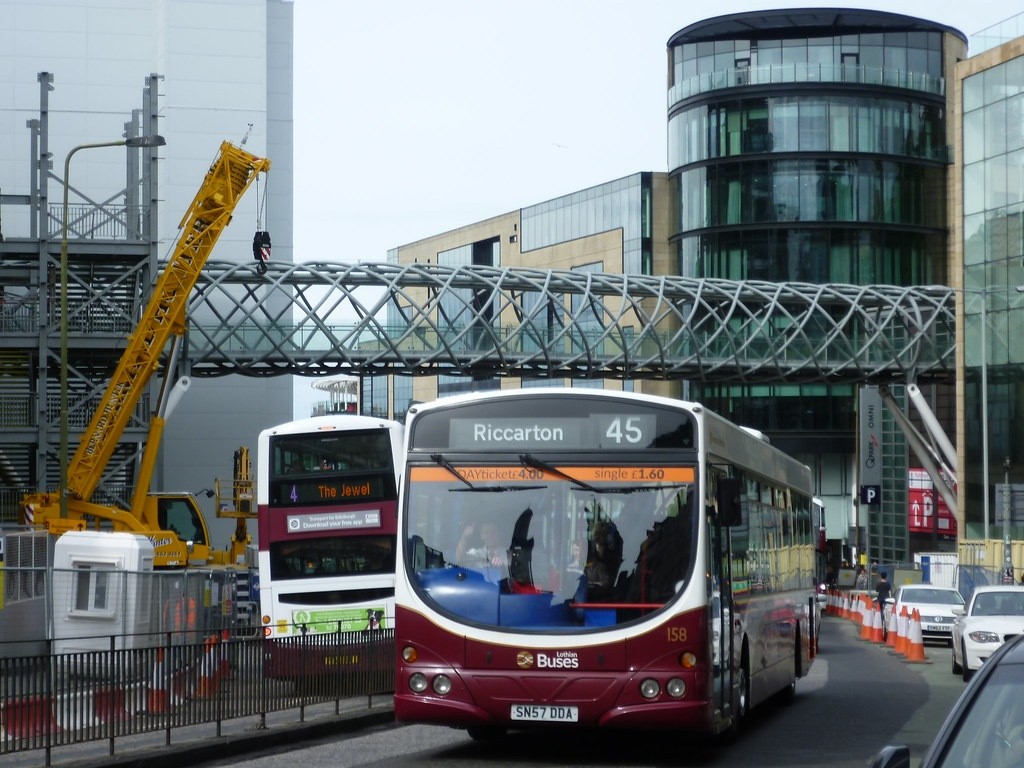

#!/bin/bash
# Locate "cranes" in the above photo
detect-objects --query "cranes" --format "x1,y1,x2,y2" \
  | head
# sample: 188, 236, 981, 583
24, 143, 273, 568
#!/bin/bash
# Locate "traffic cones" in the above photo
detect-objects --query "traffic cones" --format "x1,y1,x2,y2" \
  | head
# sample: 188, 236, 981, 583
220, 633, 231, 682
901, 610, 931, 664
140, 648, 173, 716
827, 590, 917, 658
196, 637, 219, 700
870, 602, 885, 644
842, 594, 849, 620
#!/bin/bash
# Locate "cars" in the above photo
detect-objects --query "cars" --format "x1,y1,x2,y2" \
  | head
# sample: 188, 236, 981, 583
896, 585, 966, 647
952, 586, 1024, 681
870, 634, 1024, 768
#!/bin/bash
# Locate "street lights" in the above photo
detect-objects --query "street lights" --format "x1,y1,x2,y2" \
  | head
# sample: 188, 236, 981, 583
59, 135, 166, 517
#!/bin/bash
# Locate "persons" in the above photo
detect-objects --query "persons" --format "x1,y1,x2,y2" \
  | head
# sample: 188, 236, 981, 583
455, 519, 511, 566
856, 569, 869, 590
876, 572, 891, 620
568, 541, 608, 586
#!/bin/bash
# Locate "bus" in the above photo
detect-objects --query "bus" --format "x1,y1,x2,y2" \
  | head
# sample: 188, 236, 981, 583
395, 388, 826, 750
257, 413, 404, 653
